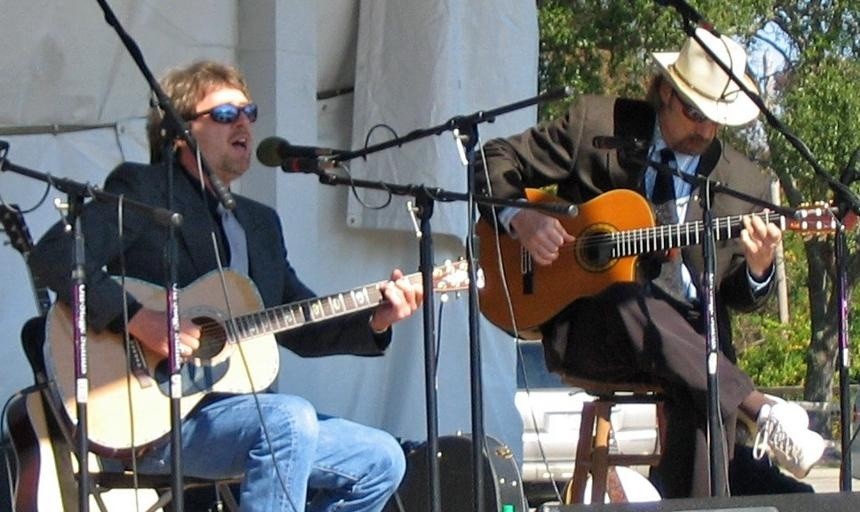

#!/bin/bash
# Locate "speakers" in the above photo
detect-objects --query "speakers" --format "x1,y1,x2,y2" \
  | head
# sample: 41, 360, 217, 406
541, 491, 860, 512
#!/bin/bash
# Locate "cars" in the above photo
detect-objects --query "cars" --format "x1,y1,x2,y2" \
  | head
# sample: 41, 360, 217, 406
514, 337, 663, 503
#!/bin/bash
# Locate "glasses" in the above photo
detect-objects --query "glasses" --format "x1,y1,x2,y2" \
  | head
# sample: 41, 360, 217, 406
673, 87, 708, 123
185, 102, 258, 125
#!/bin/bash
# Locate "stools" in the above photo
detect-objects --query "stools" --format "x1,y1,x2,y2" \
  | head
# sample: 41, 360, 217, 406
561, 373, 669, 505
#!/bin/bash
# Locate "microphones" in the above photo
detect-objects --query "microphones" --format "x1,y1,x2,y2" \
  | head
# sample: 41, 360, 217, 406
591, 134, 637, 151
257, 134, 347, 167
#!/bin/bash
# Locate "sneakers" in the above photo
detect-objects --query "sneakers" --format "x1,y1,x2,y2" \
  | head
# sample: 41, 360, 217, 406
753, 394, 825, 478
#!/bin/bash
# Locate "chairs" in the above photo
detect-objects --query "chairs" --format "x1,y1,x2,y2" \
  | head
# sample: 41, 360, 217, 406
21, 318, 243, 511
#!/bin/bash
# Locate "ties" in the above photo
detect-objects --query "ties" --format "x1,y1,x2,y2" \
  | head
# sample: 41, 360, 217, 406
651, 150, 686, 309
217, 201, 251, 275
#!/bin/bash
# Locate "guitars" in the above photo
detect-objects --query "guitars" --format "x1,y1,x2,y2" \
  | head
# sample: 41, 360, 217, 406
43, 255, 485, 459
466, 182, 859, 340
0, 200, 164, 512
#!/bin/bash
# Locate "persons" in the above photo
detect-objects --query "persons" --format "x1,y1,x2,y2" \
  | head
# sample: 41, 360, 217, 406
468, 28, 828, 497
29, 62, 425, 512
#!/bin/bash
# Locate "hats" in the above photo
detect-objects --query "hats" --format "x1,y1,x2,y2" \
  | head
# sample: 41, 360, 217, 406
653, 30, 761, 128
